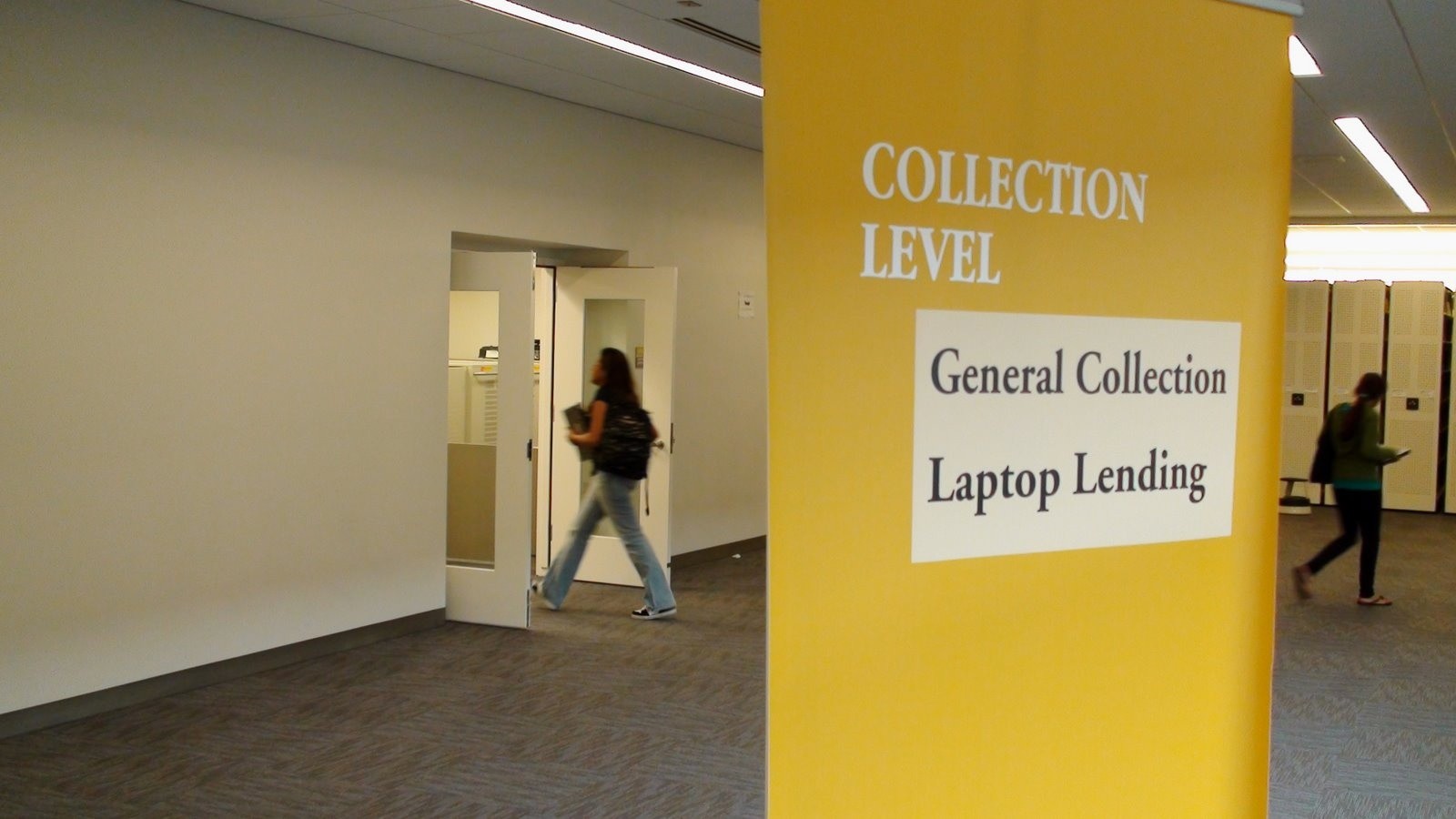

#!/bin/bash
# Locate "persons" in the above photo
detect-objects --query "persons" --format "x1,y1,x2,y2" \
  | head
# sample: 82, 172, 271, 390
531, 347, 677, 620
1292, 373, 1399, 605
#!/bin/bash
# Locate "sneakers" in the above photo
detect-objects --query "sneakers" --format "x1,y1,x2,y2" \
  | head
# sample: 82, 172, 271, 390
531, 580, 557, 610
631, 604, 676, 620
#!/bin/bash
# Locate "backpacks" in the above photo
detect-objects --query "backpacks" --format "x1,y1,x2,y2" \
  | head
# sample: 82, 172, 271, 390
592, 401, 651, 516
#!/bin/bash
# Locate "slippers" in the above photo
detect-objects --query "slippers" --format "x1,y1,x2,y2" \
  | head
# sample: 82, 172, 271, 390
1357, 595, 1392, 606
1293, 567, 1313, 598
